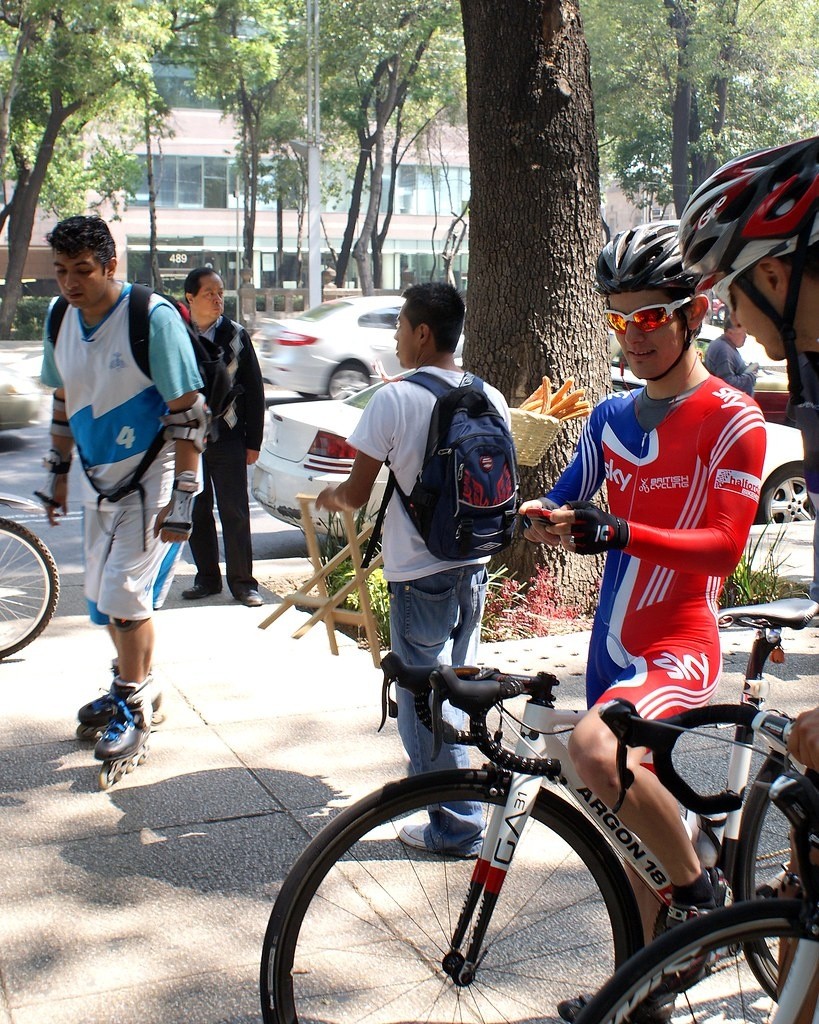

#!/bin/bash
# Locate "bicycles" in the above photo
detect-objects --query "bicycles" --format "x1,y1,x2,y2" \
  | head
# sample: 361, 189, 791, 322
1, 493, 61, 661
257, 597, 819, 1024
538, 697, 819, 1024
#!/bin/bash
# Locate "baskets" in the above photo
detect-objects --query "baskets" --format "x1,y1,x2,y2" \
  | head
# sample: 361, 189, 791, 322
508, 407, 564, 467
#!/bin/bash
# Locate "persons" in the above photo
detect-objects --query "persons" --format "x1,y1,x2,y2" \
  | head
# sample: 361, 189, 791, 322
180, 265, 265, 608
679, 133, 818, 1024
521, 223, 768, 1024
33, 214, 210, 794
315, 282, 523, 859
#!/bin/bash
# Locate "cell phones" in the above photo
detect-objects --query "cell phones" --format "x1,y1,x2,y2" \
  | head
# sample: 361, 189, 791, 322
526, 509, 557, 527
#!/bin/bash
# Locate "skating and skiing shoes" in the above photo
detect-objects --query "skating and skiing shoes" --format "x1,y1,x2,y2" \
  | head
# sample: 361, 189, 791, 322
76, 658, 167, 741
95, 674, 152, 790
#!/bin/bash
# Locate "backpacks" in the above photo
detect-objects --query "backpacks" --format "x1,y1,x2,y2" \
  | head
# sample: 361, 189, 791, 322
361, 373, 522, 562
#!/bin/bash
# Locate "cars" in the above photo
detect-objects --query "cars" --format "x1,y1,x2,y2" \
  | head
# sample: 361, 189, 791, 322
609, 294, 817, 419
250, 357, 815, 542
248, 295, 466, 402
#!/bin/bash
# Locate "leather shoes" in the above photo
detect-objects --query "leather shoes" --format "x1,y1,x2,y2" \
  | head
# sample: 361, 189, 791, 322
182, 585, 222, 600
233, 588, 264, 607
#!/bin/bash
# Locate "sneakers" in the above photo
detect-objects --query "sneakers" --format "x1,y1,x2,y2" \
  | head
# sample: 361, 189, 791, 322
667, 867, 734, 994
557, 994, 592, 1023
400, 824, 480, 858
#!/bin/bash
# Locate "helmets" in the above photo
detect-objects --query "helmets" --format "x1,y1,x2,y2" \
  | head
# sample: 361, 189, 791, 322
679, 136, 819, 294
595, 220, 702, 294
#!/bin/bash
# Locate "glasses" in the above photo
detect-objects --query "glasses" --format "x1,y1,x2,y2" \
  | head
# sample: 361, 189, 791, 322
606, 297, 691, 334
712, 240, 792, 313
